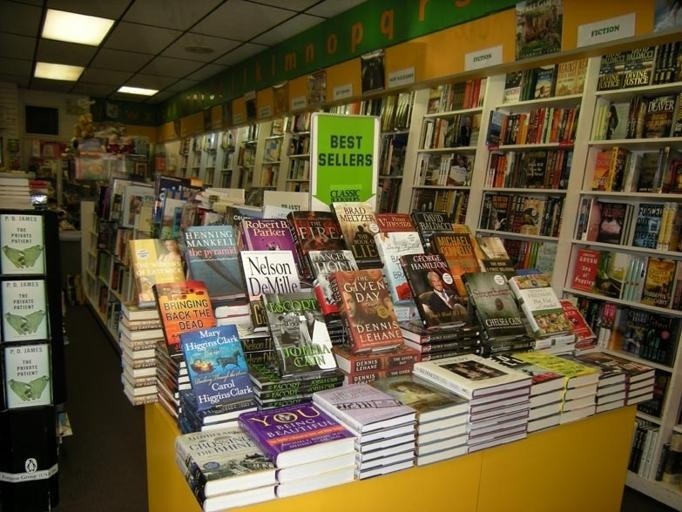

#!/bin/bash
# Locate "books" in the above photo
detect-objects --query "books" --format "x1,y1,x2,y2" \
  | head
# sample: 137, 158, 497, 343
562, 41, 682, 512
2, 136, 155, 511
119, 176, 656, 511
155, 90, 414, 215
411, 76, 488, 225
475, 57, 588, 284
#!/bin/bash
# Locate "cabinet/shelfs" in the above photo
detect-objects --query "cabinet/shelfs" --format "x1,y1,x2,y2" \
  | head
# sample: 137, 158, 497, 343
57, 29, 681, 511
